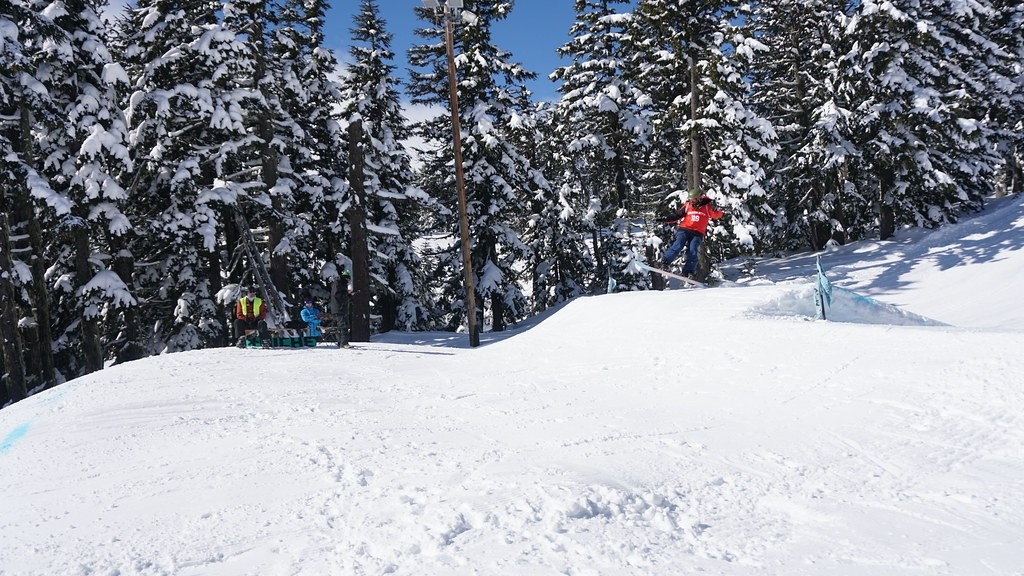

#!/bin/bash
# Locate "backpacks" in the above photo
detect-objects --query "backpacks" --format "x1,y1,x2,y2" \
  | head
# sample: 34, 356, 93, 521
285, 320, 307, 335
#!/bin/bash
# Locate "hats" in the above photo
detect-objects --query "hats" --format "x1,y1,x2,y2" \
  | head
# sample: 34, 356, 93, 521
306, 299, 312, 304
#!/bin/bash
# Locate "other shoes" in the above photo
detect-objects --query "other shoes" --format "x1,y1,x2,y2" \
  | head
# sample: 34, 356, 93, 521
236, 337, 246, 348
688, 272, 695, 280
661, 256, 670, 272
262, 339, 271, 349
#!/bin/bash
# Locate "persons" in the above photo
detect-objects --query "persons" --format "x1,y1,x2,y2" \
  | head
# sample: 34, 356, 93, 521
300, 297, 322, 342
234, 287, 272, 349
652, 189, 723, 279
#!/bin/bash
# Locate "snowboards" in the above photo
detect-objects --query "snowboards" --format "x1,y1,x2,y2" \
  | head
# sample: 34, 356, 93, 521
633, 260, 707, 288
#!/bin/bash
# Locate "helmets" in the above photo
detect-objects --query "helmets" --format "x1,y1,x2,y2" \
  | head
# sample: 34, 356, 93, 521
687, 188, 701, 199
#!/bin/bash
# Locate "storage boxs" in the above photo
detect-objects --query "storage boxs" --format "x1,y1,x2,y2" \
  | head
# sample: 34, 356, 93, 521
246, 329, 316, 348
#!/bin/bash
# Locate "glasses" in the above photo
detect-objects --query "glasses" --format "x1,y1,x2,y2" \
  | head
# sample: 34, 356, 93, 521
250, 292, 255, 293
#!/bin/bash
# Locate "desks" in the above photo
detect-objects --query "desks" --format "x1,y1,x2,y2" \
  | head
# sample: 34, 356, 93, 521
316, 326, 351, 347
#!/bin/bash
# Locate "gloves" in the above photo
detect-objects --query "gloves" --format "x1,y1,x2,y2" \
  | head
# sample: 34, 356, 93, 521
692, 199, 712, 208
651, 216, 667, 222
